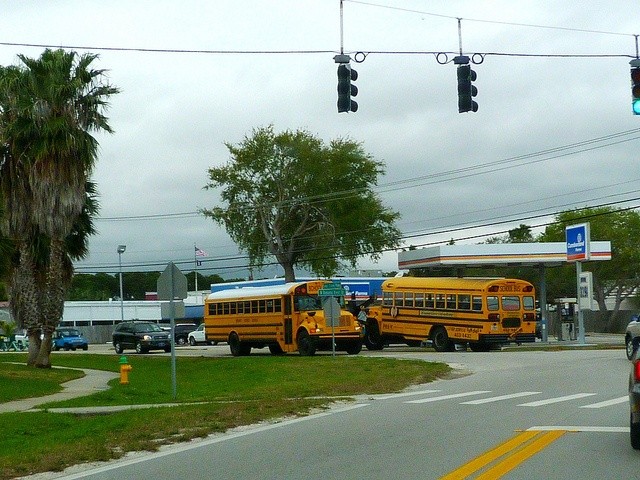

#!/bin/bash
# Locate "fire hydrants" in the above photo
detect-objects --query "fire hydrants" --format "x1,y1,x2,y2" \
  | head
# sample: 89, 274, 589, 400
119, 354, 132, 384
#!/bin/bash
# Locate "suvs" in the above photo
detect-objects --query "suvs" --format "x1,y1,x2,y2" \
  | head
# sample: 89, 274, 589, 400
112, 319, 171, 353
187, 322, 217, 345
625, 313, 640, 360
174, 324, 198, 344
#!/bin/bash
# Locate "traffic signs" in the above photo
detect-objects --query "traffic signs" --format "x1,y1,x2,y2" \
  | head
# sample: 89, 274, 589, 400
318, 289, 346, 296
322, 282, 340, 288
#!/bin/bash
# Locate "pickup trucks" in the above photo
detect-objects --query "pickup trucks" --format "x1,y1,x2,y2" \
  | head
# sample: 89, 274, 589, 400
14, 326, 54, 350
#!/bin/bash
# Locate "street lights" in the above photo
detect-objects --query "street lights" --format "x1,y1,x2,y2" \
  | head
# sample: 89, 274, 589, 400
117, 244, 126, 321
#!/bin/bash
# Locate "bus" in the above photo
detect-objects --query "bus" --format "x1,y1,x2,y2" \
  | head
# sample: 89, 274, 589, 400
365, 276, 535, 351
203, 280, 365, 355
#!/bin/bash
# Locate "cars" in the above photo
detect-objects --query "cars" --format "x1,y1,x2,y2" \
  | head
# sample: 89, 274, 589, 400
629, 346, 640, 449
52, 326, 87, 350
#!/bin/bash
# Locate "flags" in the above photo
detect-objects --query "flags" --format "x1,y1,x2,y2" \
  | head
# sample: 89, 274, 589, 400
195, 247, 208, 256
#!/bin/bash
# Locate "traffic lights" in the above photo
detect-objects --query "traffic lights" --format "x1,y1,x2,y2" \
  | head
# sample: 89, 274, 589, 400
457, 63, 477, 112
631, 67, 640, 115
336, 62, 357, 112
